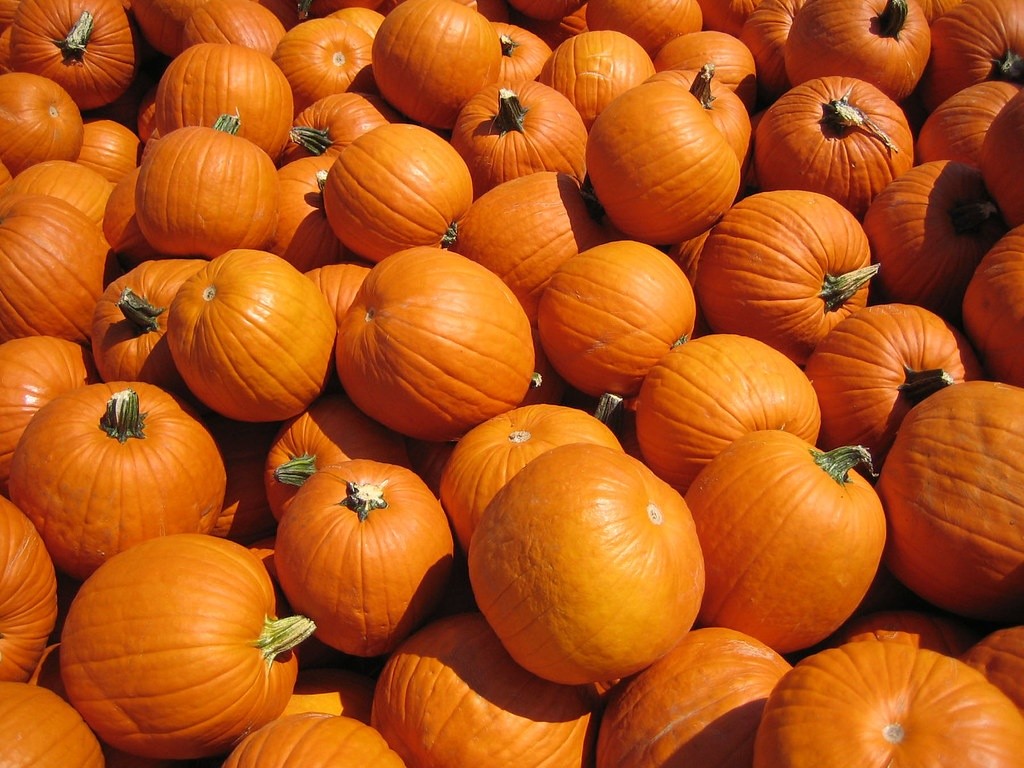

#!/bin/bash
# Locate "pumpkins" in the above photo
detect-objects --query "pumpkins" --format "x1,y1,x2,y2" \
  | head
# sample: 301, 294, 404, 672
2, 0, 1024, 767
